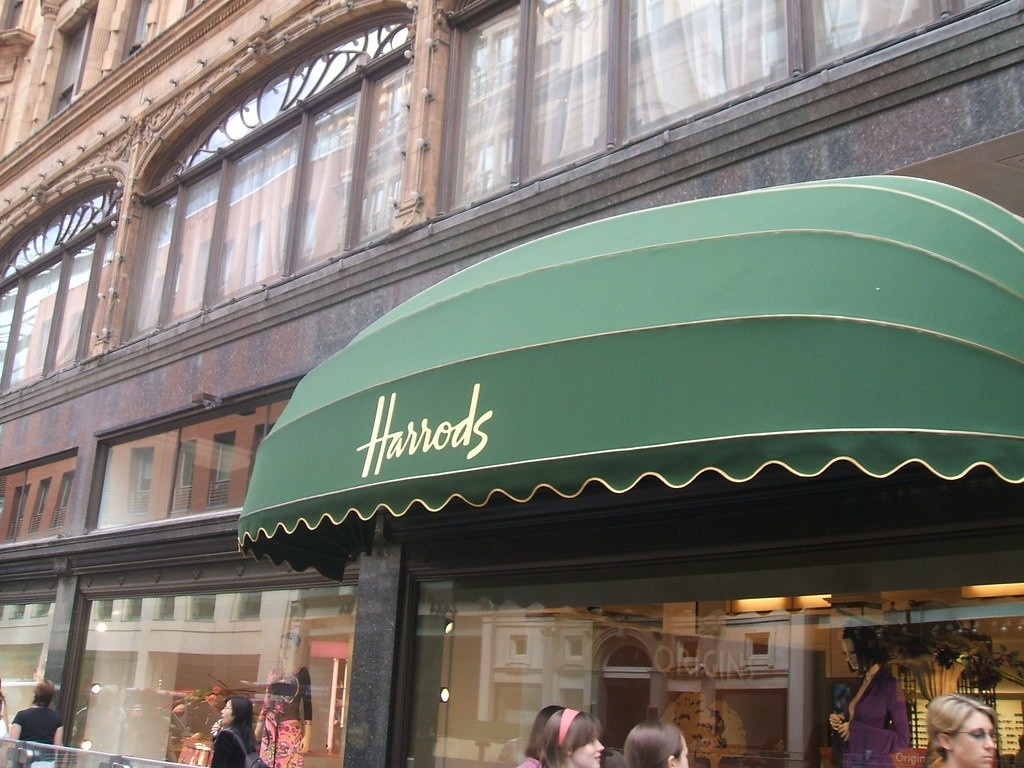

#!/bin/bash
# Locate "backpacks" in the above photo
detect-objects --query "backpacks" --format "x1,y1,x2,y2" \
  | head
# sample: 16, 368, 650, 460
213, 729, 271, 768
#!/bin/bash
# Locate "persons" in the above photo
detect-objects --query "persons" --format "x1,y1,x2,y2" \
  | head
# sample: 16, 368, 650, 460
254, 632, 312, 768
9, 680, 64, 767
0, 677, 9, 746
764, 735, 786, 768
922, 692, 1000, 768
623, 719, 689, 768
210, 695, 257, 768
70, 682, 168, 768
827, 623, 910, 768
516, 704, 604, 768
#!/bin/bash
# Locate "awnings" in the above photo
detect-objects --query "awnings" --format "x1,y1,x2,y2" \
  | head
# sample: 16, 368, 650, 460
237, 175, 1024, 584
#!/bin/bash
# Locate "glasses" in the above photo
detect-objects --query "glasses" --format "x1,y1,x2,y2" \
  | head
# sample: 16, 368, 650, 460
944, 728, 998, 743
